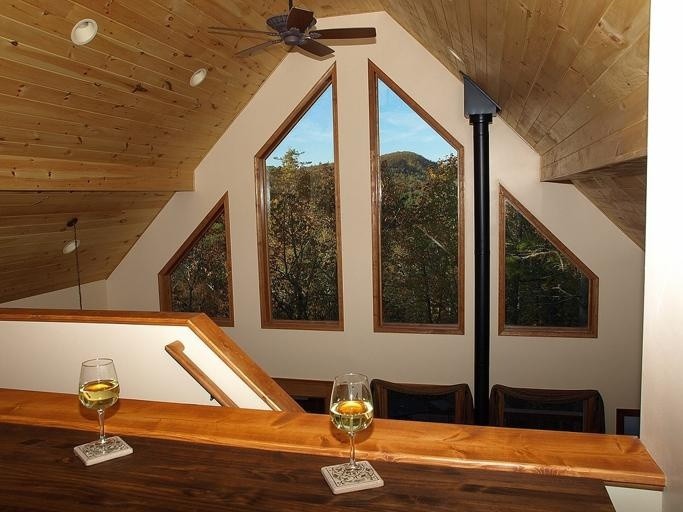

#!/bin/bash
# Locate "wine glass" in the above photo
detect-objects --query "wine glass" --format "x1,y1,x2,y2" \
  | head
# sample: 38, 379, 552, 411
321, 371, 385, 495
74, 358, 135, 468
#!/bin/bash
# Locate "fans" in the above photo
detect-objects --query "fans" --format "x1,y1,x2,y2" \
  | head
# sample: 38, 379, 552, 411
208, 1, 376, 57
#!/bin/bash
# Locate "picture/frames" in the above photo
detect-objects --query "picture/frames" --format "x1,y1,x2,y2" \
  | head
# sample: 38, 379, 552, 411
490, 384, 606, 435
615, 408, 639, 435
369, 379, 474, 425
272, 378, 348, 416
